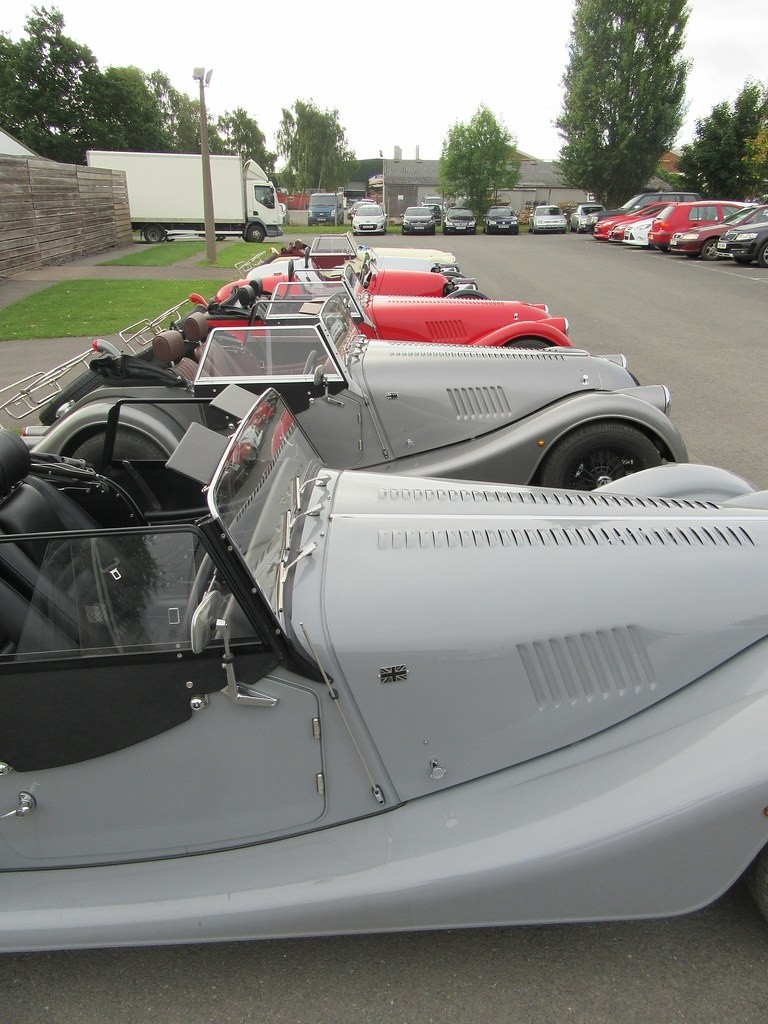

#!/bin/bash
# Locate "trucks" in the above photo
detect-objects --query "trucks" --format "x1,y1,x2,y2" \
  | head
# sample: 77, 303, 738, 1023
85, 151, 284, 242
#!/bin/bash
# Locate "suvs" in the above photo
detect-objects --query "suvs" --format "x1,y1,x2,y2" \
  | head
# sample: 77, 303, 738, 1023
586, 192, 708, 236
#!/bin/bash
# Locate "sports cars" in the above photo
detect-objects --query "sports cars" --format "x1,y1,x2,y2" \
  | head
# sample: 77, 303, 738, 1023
183, 231, 572, 350
2, 390, 768, 952
0, 293, 690, 496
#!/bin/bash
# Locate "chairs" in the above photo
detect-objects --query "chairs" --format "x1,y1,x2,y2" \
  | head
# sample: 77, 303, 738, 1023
701, 212, 715, 220
238, 284, 267, 321
249, 278, 271, 308
0, 431, 192, 656
302, 248, 326, 287
288, 259, 308, 300
153, 331, 211, 382
185, 311, 248, 377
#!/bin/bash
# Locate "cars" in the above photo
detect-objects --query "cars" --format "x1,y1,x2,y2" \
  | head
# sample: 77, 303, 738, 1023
351, 205, 388, 235
529, 205, 568, 233
670, 204, 768, 260
647, 201, 756, 256
346, 198, 382, 224
592, 201, 758, 256
420, 194, 450, 223
716, 208, 768, 267
570, 204, 607, 234
483, 206, 521, 233
442, 207, 478, 234
400, 207, 437, 236
278, 203, 291, 226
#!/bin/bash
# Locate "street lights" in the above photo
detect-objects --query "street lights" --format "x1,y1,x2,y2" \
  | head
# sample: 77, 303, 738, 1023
192, 66, 217, 260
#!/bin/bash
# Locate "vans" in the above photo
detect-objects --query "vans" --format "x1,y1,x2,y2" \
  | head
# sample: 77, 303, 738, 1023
304, 192, 345, 226
